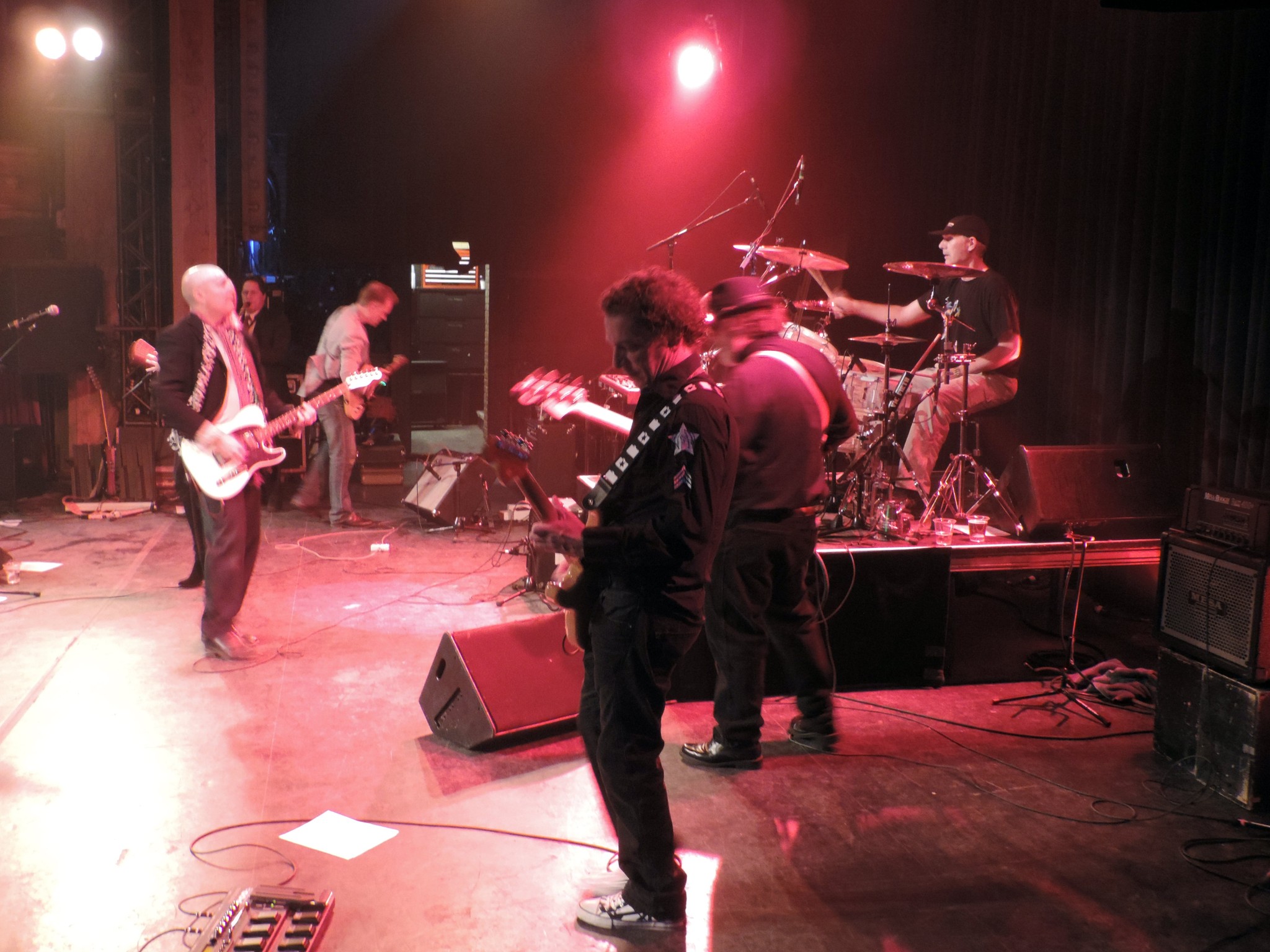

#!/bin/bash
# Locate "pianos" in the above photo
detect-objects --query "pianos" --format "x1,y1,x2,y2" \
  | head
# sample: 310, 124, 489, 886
598, 373, 641, 405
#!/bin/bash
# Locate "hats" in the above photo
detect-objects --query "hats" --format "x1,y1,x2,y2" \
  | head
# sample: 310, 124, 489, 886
709, 277, 783, 318
929, 214, 989, 247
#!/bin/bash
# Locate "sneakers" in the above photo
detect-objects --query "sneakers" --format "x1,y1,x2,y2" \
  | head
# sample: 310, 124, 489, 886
576, 891, 685, 932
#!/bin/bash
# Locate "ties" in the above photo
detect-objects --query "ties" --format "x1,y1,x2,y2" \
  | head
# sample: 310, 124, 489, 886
242, 315, 252, 335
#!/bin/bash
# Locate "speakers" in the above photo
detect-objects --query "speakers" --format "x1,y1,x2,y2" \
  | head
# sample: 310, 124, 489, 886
400, 448, 496, 525
1156, 542, 1263, 682
418, 610, 584, 755
1152, 645, 1270, 811
984, 445, 1176, 537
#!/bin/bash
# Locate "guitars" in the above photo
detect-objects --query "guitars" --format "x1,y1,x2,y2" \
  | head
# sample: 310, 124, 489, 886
181, 366, 383, 500
484, 429, 606, 652
508, 366, 641, 437
343, 354, 410, 421
86, 365, 117, 497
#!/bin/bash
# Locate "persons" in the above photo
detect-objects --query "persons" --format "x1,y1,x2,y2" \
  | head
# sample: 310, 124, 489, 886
680, 277, 860, 771
161, 264, 317, 661
146, 351, 208, 588
241, 275, 290, 513
829, 215, 1020, 510
290, 280, 399, 526
529, 266, 740, 933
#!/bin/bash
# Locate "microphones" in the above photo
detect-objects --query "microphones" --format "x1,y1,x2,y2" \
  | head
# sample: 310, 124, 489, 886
846, 348, 866, 372
1065, 532, 1095, 541
7, 304, 61, 329
419, 458, 442, 481
750, 174, 772, 212
934, 367, 941, 403
794, 160, 806, 207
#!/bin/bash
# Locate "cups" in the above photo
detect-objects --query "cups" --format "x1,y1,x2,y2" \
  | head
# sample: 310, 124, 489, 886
932, 518, 957, 545
966, 515, 990, 542
3, 561, 21, 584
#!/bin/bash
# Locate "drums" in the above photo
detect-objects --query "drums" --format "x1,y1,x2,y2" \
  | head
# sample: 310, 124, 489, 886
776, 321, 839, 366
836, 368, 914, 420
833, 355, 885, 453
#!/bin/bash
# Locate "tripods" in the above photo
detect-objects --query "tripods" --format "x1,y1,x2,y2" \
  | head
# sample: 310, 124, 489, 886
991, 539, 1120, 728
816, 346, 936, 544
911, 337, 1022, 537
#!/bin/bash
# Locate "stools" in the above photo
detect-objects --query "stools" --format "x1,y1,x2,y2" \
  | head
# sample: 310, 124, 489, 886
954, 399, 1015, 509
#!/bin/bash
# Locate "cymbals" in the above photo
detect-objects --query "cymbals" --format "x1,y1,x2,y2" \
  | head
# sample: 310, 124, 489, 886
847, 333, 928, 346
883, 261, 989, 279
792, 300, 838, 313
733, 244, 849, 271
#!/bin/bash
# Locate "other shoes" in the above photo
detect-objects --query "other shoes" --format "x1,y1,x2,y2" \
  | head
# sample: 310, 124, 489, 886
179, 562, 204, 587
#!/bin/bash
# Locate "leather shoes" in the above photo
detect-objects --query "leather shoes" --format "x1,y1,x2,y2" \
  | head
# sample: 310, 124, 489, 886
679, 739, 765, 769
330, 514, 372, 528
205, 633, 260, 662
789, 714, 837, 746
